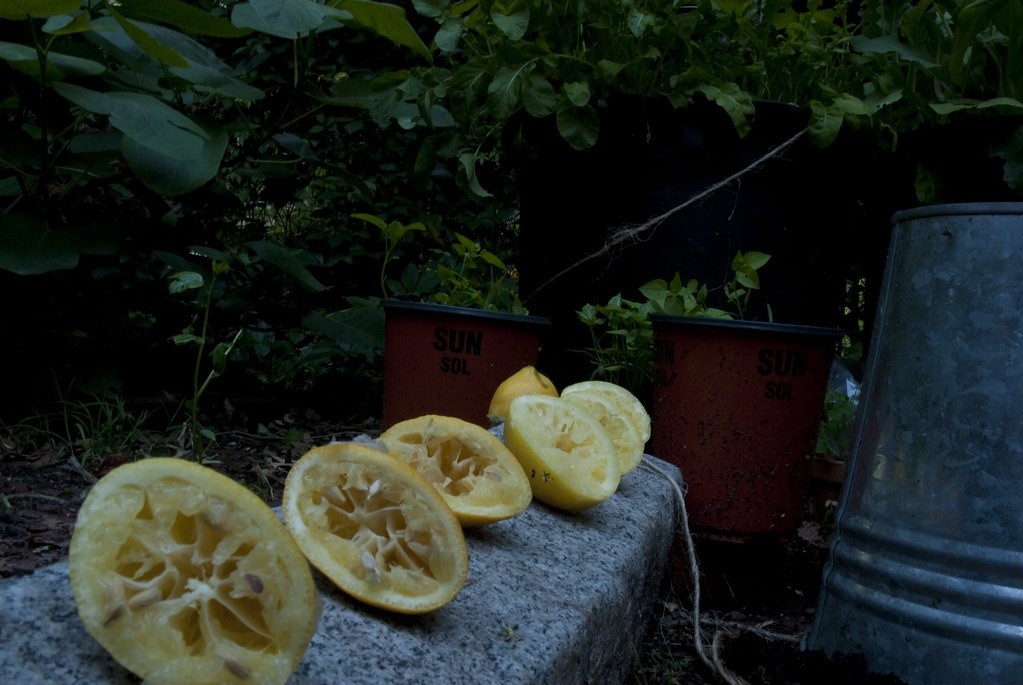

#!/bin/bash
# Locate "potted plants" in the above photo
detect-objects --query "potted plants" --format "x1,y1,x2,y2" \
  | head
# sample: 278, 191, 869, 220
646, 248, 846, 534
408, 0, 1023, 371
350, 200, 552, 442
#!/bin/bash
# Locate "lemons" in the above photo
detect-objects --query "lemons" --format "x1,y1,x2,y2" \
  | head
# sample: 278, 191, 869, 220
69, 362, 652, 685
